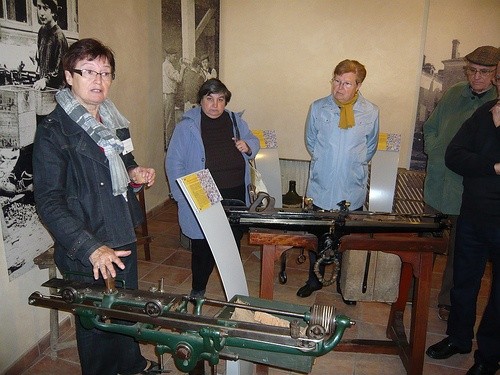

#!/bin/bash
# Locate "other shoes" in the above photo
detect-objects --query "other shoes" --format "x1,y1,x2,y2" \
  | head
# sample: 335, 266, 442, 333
142, 360, 164, 375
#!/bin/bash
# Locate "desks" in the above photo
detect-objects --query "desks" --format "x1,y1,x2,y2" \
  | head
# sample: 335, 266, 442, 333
248, 228, 450, 375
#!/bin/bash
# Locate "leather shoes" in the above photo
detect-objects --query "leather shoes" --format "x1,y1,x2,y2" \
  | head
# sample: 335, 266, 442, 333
297, 284, 320, 297
426, 335, 472, 359
466, 362, 495, 375
337, 288, 356, 305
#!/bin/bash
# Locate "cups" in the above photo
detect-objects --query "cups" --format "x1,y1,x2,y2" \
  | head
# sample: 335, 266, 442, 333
134, 166, 151, 190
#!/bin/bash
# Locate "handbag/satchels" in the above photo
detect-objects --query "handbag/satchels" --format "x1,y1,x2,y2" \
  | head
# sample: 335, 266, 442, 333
232, 111, 268, 208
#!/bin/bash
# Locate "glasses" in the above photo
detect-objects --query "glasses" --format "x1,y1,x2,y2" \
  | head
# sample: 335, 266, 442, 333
466, 66, 497, 76
68, 68, 116, 81
331, 76, 361, 87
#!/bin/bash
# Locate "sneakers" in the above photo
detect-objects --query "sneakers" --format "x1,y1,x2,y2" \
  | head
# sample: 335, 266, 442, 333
438, 305, 451, 321
406, 297, 413, 305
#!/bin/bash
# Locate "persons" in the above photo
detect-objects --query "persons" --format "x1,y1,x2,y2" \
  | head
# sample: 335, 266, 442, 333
165, 77, 260, 302
297, 59, 380, 306
406, 46, 500, 323
31, 0, 69, 91
196, 54, 217, 83
164, 48, 186, 155
33, 38, 163, 375
426, 97, 500, 375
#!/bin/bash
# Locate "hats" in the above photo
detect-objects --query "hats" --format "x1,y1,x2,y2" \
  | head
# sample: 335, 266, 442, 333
465, 46, 500, 66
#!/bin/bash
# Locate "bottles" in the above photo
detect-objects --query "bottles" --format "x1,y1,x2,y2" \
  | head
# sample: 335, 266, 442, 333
282, 181, 303, 209
